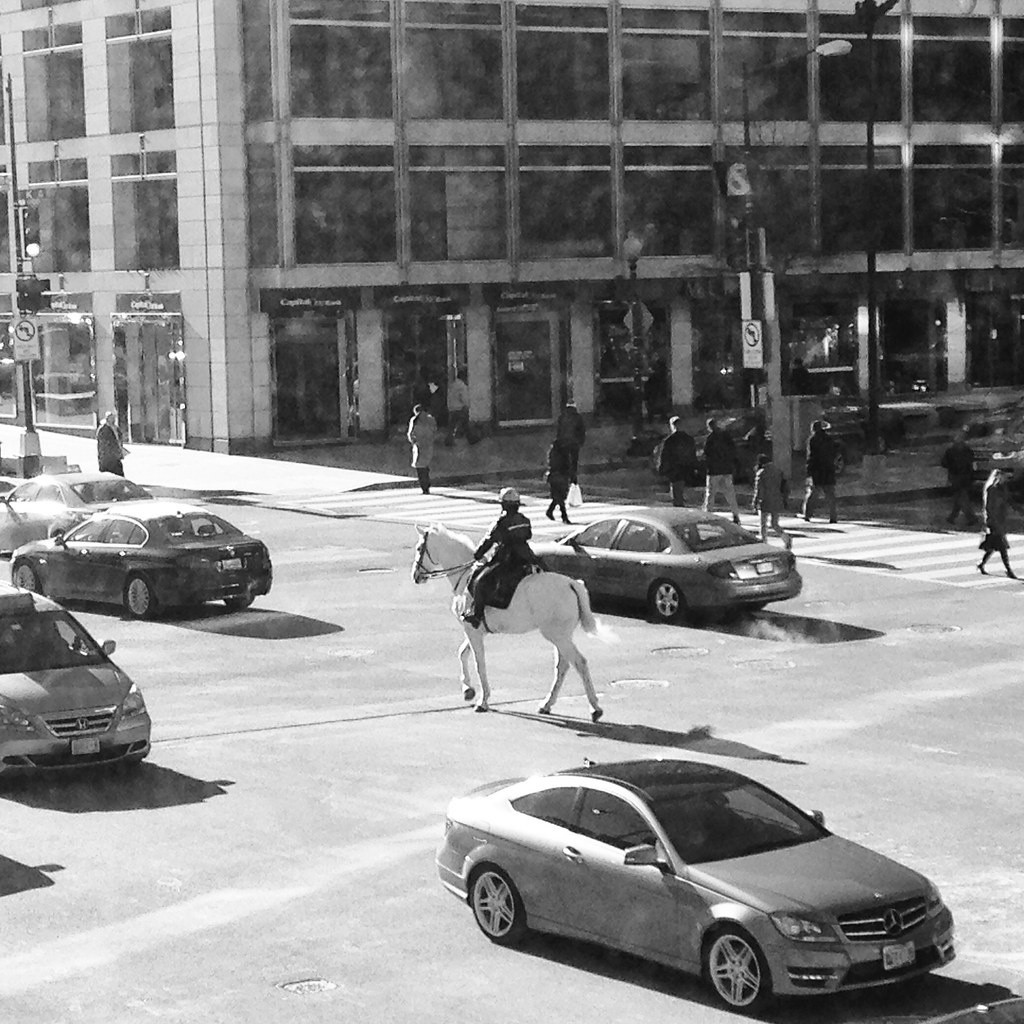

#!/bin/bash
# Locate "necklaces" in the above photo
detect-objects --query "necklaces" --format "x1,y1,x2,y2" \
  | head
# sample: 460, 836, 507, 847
941, 434, 979, 525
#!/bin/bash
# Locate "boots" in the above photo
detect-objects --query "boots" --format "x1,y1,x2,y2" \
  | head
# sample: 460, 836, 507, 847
561, 511, 572, 525
546, 504, 556, 520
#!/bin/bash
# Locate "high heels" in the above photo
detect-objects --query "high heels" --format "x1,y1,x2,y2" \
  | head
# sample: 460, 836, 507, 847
1005, 571, 1017, 579
977, 564, 988, 574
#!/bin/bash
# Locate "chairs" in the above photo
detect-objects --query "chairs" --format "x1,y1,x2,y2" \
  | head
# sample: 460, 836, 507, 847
199, 525, 216, 536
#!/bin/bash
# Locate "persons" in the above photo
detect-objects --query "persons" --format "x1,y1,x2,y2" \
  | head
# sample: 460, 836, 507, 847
698, 419, 742, 524
796, 420, 838, 523
544, 397, 584, 524
463, 487, 533, 630
788, 356, 810, 393
753, 455, 792, 549
95, 411, 126, 478
657, 415, 700, 507
408, 405, 436, 494
444, 370, 480, 447
975, 469, 1017, 578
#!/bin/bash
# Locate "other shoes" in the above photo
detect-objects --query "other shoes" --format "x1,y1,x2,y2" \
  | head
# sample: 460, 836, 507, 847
463, 613, 481, 630
783, 533, 793, 550
797, 513, 809, 521
732, 517, 741, 525
830, 519, 837, 523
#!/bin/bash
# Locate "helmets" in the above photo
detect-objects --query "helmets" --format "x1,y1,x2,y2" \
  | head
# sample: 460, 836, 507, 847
497, 487, 525, 507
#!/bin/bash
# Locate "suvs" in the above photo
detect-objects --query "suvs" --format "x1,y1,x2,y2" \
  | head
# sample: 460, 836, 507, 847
0, 578, 153, 785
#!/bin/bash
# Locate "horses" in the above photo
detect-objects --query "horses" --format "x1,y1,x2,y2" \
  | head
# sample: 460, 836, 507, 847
411, 523, 604, 723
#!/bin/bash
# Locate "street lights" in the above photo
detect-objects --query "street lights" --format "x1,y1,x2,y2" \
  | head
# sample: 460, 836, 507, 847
741, 39, 852, 418
621, 231, 654, 459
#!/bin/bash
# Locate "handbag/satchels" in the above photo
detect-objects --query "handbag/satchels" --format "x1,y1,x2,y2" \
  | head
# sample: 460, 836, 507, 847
780, 469, 791, 496
544, 470, 552, 486
567, 483, 583, 508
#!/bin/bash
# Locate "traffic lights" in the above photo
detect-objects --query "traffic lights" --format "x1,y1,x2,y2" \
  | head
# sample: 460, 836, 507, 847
23, 207, 41, 257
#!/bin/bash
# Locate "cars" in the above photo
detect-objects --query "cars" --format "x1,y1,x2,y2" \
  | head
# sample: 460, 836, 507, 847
963, 414, 1024, 483
8, 502, 274, 622
434, 758, 956, 1009
0, 470, 156, 554
530, 507, 804, 621
652, 409, 867, 488
